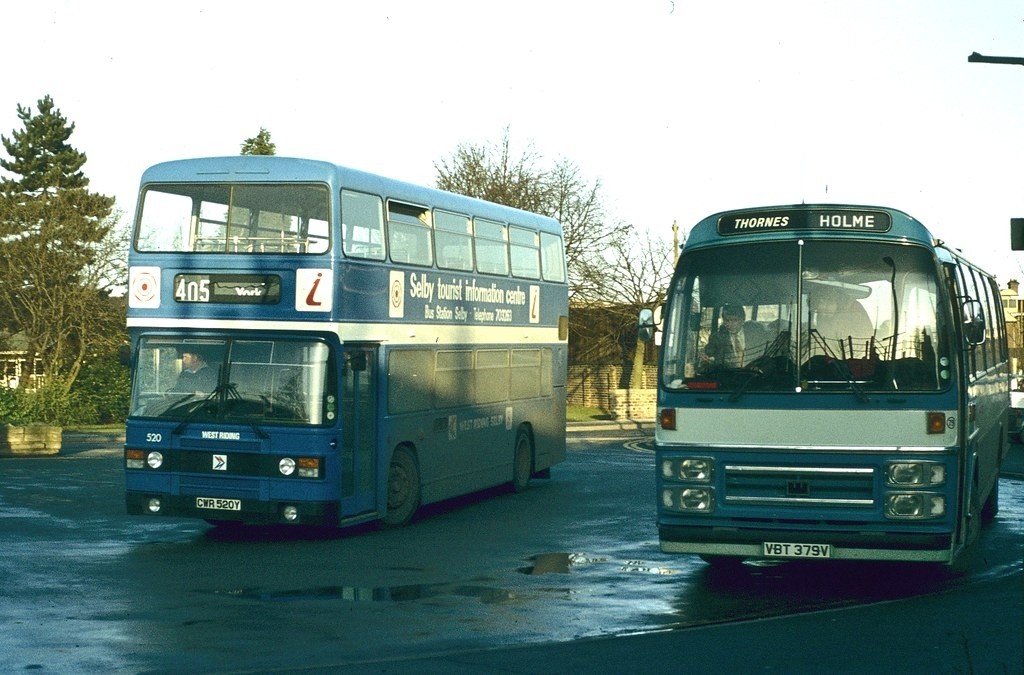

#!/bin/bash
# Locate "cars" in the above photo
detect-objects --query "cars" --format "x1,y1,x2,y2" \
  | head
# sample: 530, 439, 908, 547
1009, 374, 1024, 444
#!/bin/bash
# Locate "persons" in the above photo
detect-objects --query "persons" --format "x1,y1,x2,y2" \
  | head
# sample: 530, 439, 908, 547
804, 286, 874, 378
165, 346, 220, 397
692, 303, 773, 373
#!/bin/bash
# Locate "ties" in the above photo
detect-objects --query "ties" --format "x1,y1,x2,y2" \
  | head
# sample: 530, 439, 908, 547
729, 330, 745, 364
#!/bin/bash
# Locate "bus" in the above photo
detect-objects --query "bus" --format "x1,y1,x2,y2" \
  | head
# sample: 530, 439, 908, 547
117, 155, 571, 535
639, 199, 1011, 579
